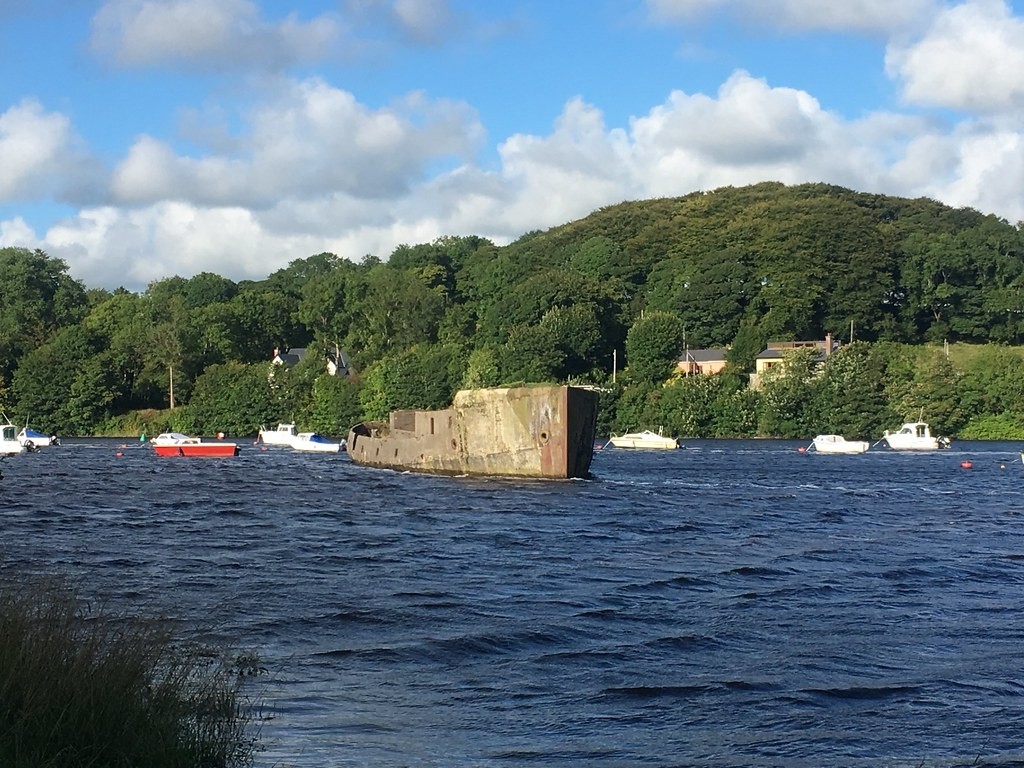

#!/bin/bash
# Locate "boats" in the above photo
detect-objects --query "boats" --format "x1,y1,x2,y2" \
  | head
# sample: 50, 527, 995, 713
16, 416, 62, 447
150, 437, 239, 457
258, 418, 298, 445
150, 431, 202, 445
883, 405, 951, 450
346, 381, 601, 482
812, 434, 871, 455
608, 425, 681, 449
284, 431, 347, 454
0, 410, 42, 456
